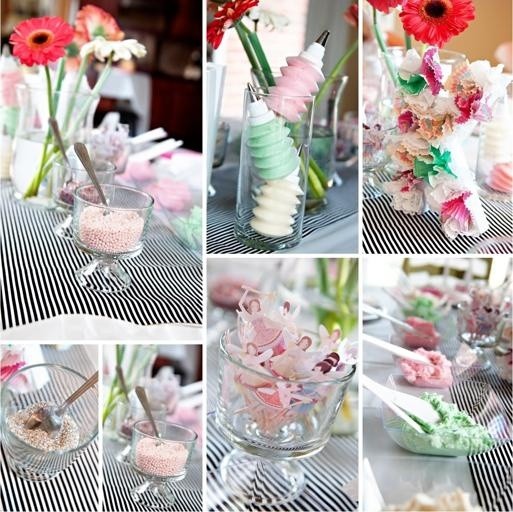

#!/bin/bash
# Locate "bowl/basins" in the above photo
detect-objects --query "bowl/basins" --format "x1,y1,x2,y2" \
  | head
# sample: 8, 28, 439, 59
390, 307, 467, 349
378, 369, 513, 459
362, 455, 513, 512
374, 328, 493, 388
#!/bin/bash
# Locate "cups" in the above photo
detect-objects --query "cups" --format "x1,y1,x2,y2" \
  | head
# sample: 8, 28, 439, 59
232, 88, 316, 250
0, 361, 106, 482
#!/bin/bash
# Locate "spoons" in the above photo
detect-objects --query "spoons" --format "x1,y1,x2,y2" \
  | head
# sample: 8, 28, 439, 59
22, 368, 99, 435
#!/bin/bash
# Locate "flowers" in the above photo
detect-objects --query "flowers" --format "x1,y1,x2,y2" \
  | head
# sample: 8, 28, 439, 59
207, 0, 358, 204
9, 4, 146, 200
366, 0, 476, 98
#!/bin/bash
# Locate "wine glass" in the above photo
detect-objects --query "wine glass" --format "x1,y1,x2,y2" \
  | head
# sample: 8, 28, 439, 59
216, 316, 356, 506
126, 419, 200, 508
68, 182, 156, 294
362, 108, 405, 203
48, 154, 117, 243
453, 303, 507, 371
109, 398, 169, 470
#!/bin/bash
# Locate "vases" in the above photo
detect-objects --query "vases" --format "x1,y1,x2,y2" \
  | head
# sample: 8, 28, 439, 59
377, 46, 471, 127
102, 344, 158, 441
271, 257, 359, 436
252, 68, 349, 209
9, 82, 105, 205
